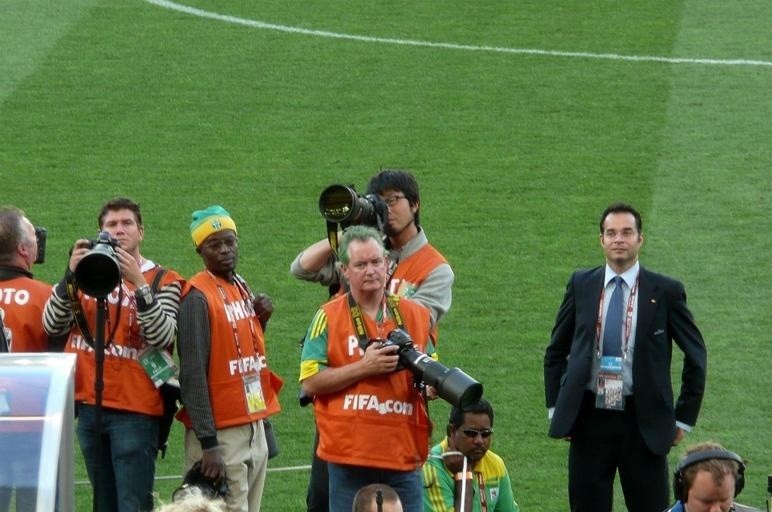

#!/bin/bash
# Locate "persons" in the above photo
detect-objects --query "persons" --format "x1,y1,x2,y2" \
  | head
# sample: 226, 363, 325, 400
542, 201, 708, 512
419, 397, 520, 511
39, 196, 186, 512
1, 203, 61, 511
354, 477, 406, 511
290, 169, 455, 511
299, 223, 443, 511
664, 440, 764, 512
174, 203, 284, 512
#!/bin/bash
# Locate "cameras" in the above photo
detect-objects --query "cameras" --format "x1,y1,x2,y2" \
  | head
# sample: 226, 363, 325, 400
377, 328, 484, 412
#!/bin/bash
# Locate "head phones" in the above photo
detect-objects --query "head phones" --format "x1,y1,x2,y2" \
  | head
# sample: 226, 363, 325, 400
672, 448, 747, 503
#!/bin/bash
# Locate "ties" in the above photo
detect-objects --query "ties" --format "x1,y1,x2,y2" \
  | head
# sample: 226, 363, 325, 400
603, 276, 624, 356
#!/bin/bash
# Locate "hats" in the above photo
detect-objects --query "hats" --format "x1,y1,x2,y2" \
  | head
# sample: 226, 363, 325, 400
190, 206, 238, 250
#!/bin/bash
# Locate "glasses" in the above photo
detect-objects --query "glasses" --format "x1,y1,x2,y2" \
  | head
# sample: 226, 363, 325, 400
463, 429, 494, 438
385, 196, 408, 207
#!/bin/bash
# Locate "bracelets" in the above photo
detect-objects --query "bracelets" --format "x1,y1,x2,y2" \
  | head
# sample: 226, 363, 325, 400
133, 285, 151, 297
